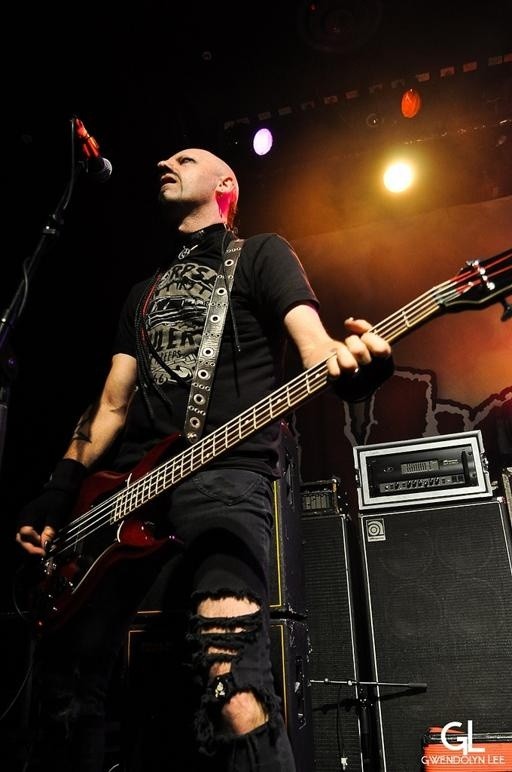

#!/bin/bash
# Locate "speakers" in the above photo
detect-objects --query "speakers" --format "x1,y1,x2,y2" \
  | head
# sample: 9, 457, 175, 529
358, 499, 512, 770
294, 515, 363, 771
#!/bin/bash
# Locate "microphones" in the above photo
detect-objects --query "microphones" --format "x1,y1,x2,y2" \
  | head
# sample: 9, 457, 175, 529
70, 115, 112, 181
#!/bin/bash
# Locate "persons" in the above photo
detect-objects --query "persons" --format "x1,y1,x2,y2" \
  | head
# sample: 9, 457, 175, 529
3, 146, 393, 772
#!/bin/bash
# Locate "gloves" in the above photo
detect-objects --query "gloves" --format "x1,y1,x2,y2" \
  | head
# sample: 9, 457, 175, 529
22, 457, 88, 533
326, 350, 395, 404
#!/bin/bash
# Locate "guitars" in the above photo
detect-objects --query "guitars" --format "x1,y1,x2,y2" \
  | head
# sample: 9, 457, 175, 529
0, 249, 512, 638
